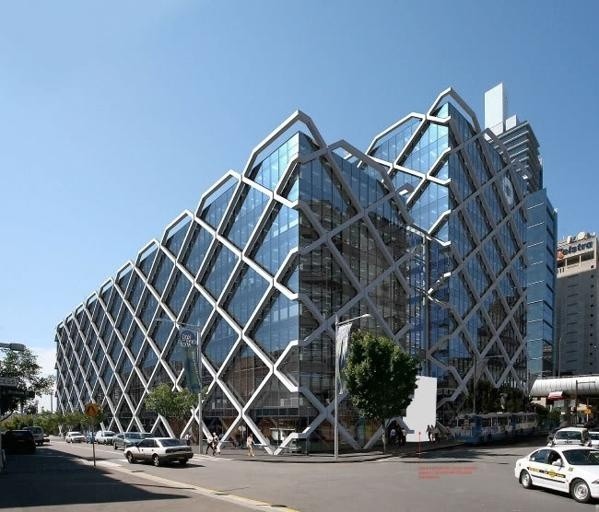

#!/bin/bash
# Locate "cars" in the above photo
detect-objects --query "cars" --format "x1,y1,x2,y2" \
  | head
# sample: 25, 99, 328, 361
64, 430, 194, 467
514, 426, 599, 503
0, 426, 51, 454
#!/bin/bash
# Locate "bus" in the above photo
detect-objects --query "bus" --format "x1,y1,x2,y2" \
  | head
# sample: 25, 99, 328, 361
449, 410, 540, 447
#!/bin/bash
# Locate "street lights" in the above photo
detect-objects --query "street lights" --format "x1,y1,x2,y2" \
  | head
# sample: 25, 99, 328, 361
154, 317, 204, 457
333, 313, 373, 460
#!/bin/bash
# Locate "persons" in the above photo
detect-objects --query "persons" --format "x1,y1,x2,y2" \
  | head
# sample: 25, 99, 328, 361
205, 432, 215, 454
390, 426, 408, 449
185, 433, 193, 446
246, 433, 255, 456
212, 432, 221, 455
426, 425, 452, 444
